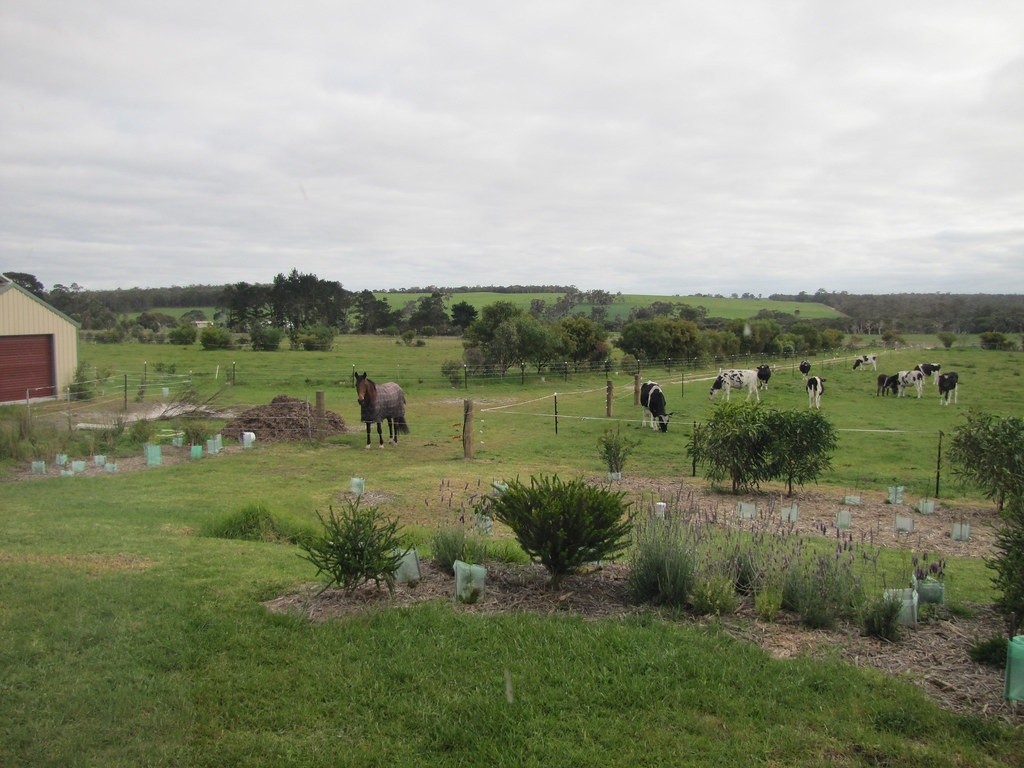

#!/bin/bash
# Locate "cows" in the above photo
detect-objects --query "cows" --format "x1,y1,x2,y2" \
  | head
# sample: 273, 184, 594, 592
639, 379, 674, 433
799, 359, 812, 381
877, 363, 959, 406
708, 369, 760, 404
756, 364, 771, 390
805, 376, 827, 410
852, 355, 877, 372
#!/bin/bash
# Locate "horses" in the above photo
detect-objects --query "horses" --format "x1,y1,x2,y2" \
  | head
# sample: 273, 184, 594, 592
355, 371, 411, 449
